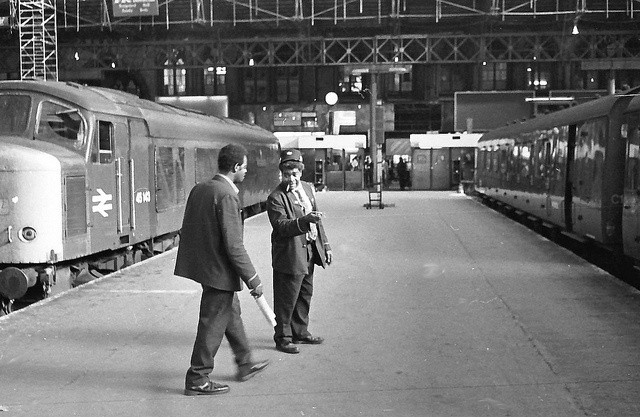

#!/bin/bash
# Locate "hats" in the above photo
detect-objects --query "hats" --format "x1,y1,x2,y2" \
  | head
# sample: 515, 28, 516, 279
280, 150, 302, 162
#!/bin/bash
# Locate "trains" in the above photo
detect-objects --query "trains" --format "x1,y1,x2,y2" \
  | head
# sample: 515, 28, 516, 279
1, 78, 281, 308
475, 92, 638, 281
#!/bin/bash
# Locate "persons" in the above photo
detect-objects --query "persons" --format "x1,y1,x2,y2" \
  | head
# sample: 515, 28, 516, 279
266, 148, 332, 353
173, 144, 279, 397
395, 158, 410, 190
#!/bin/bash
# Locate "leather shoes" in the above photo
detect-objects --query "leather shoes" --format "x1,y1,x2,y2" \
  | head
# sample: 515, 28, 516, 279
238, 361, 270, 381
184, 382, 230, 396
276, 342, 296, 352
295, 336, 323, 344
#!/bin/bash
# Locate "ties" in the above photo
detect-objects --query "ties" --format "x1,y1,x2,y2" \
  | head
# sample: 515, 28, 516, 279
294, 190, 318, 242
